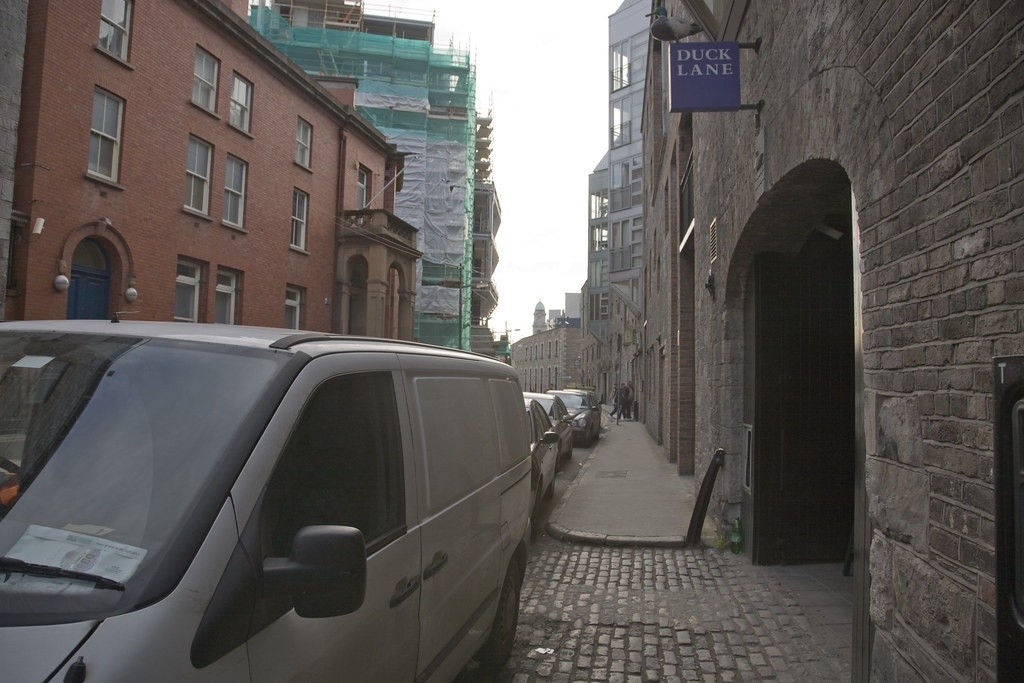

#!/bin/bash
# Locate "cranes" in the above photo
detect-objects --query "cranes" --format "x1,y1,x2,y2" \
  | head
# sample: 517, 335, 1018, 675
492, 320, 521, 342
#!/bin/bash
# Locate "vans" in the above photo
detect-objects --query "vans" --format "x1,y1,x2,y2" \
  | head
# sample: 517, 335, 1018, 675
0, 319, 532, 683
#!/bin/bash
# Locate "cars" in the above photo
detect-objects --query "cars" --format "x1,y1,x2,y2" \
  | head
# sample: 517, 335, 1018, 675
545, 389, 600, 447
523, 397, 559, 527
562, 387, 603, 431
523, 391, 574, 473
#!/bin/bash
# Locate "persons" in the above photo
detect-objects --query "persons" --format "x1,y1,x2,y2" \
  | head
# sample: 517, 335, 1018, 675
543, 382, 555, 393
610, 381, 636, 422
